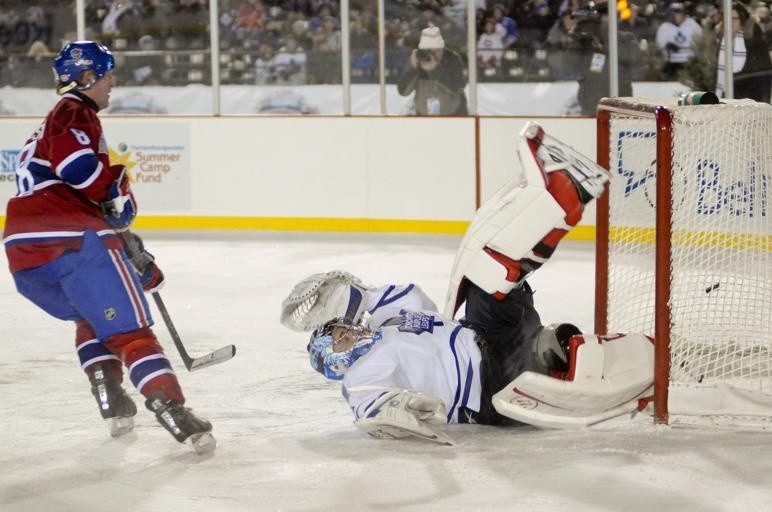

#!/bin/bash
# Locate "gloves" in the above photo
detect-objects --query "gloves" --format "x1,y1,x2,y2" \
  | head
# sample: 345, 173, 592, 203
101, 163, 136, 233
126, 237, 167, 294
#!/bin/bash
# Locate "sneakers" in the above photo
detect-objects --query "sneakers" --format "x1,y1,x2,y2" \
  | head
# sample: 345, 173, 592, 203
538, 141, 604, 200
144, 393, 211, 441
88, 365, 137, 418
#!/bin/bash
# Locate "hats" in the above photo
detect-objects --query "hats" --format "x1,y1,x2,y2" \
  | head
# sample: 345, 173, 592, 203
418, 27, 445, 53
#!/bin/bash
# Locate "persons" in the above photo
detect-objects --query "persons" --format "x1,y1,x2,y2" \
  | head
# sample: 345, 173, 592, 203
0, 0, 772, 87
702, 2, 772, 102
577, 6, 633, 117
279, 118, 654, 447
397, 26, 468, 115
4, 41, 212, 442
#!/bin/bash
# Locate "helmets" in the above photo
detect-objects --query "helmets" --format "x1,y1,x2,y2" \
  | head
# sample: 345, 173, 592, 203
52, 40, 113, 94
306, 319, 380, 381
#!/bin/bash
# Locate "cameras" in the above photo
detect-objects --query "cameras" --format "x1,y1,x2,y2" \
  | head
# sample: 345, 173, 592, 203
416, 49, 435, 61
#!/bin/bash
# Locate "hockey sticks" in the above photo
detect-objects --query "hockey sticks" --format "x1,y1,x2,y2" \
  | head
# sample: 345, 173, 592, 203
122, 230, 235, 372
